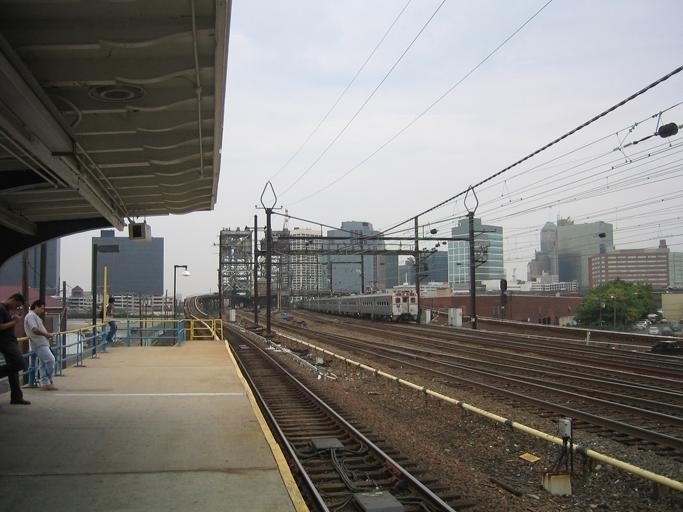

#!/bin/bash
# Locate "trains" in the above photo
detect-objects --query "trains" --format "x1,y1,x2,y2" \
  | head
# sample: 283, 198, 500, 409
289, 296, 417, 322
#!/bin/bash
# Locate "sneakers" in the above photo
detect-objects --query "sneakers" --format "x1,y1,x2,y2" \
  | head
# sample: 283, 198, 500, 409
10, 399, 31, 404
40, 383, 58, 391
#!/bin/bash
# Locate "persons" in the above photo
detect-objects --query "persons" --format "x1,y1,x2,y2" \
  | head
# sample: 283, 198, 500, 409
104, 297, 118, 342
23, 300, 57, 390
1, 293, 31, 404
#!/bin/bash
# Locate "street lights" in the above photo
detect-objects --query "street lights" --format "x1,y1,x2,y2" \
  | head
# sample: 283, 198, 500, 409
173, 264, 192, 343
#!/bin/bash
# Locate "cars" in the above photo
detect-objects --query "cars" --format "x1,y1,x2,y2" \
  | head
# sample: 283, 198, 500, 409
637, 313, 683, 337
